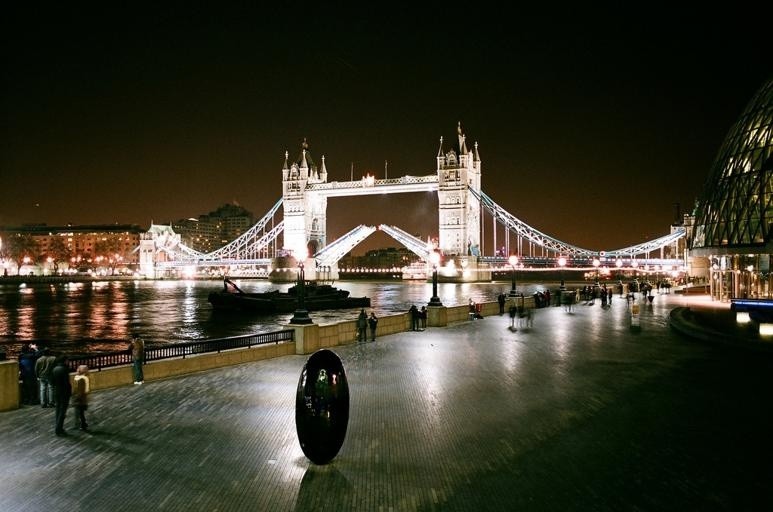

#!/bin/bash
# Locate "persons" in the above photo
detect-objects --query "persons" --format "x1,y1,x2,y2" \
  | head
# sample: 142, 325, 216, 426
408, 305, 428, 331
35, 347, 56, 408
72, 364, 90, 430
128, 333, 144, 385
356, 309, 368, 342
469, 298, 476, 320
51, 355, 72, 437
368, 313, 378, 341
18, 343, 42, 405
498, 279, 670, 328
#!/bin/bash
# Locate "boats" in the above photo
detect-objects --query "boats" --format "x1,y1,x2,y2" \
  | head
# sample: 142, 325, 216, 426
400, 261, 426, 280
206, 263, 373, 314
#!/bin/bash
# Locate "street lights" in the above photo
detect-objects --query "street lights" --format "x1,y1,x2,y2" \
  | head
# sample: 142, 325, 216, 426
286, 239, 314, 324
590, 258, 686, 288
556, 255, 568, 291
504, 253, 523, 299
426, 250, 443, 307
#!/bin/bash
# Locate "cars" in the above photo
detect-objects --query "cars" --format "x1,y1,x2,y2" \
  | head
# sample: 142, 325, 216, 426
77, 266, 134, 277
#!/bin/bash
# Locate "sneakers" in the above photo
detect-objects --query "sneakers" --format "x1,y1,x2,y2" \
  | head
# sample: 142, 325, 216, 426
134, 380, 144, 385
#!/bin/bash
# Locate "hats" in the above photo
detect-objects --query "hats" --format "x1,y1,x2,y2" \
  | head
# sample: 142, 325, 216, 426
77, 365, 89, 373
